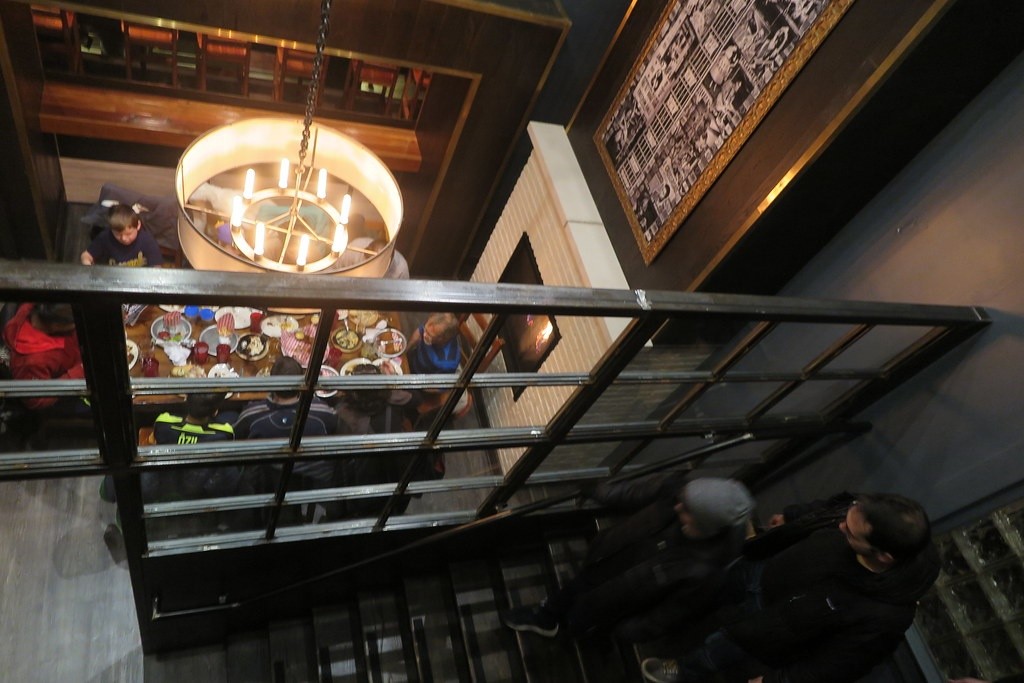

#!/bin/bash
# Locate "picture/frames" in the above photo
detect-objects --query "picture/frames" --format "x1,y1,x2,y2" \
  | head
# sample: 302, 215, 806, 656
593, 0, 852, 264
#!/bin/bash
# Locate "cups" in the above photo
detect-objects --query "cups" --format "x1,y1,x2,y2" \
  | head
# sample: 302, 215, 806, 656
217, 345, 230, 363
193, 342, 209, 366
143, 358, 159, 376
185, 305, 199, 323
200, 309, 215, 329
329, 348, 342, 368
249, 312, 265, 331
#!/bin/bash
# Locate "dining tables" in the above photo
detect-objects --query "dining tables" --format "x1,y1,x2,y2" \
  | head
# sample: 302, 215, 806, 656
123, 305, 409, 422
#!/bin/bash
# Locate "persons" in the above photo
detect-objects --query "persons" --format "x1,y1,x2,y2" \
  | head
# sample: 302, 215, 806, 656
716, 9, 789, 107
336, 237, 409, 280
505, 473, 751, 648
406, 312, 462, 373
154, 392, 235, 445
606, 108, 632, 166
232, 356, 338, 493
334, 363, 412, 436
653, 184, 670, 213
641, 490, 941, 683
80, 204, 164, 268
2, 302, 91, 416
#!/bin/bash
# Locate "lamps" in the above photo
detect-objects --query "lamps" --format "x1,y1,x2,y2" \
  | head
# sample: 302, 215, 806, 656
173, 1, 403, 315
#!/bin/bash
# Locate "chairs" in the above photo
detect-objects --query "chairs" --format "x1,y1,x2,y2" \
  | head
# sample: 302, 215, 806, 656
272, 47, 329, 109
122, 21, 178, 92
195, 31, 250, 96
343, 59, 399, 116
95, 185, 182, 269
403, 312, 505, 430
29, 4, 83, 79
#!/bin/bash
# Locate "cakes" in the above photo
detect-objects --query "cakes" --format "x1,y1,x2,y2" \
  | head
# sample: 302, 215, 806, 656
380, 332, 403, 353
281, 324, 317, 365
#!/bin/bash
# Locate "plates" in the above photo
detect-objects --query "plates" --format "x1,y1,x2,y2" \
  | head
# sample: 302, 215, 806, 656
235, 333, 269, 360
340, 358, 372, 376
208, 364, 235, 398
199, 325, 239, 357
373, 358, 402, 376
126, 339, 138, 369
316, 365, 340, 398
262, 315, 299, 338
159, 304, 186, 314
347, 310, 378, 326
331, 327, 363, 354
151, 315, 192, 344
280, 342, 330, 368
375, 328, 407, 357
215, 306, 262, 329
